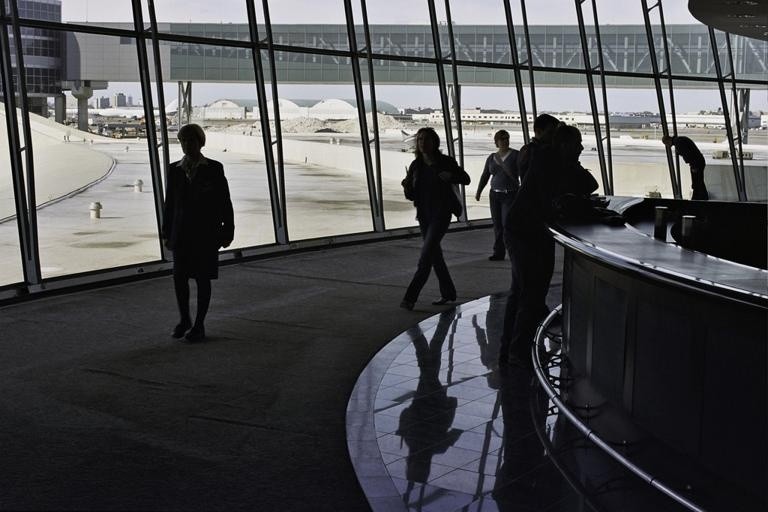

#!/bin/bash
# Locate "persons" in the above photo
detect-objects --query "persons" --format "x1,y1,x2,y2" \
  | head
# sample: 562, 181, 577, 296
516, 114, 611, 324
400, 128, 471, 311
399, 293, 564, 512
499, 121, 598, 369
662, 136, 709, 200
475, 131, 519, 261
161, 124, 235, 340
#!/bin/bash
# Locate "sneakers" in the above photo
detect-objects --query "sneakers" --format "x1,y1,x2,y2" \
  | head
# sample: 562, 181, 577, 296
430, 292, 460, 307
398, 299, 417, 312
487, 250, 508, 265
170, 319, 207, 344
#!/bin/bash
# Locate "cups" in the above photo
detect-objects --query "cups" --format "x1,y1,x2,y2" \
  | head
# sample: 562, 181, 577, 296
653, 226, 667, 241
654, 205, 668, 228
680, 215, 696, 235
